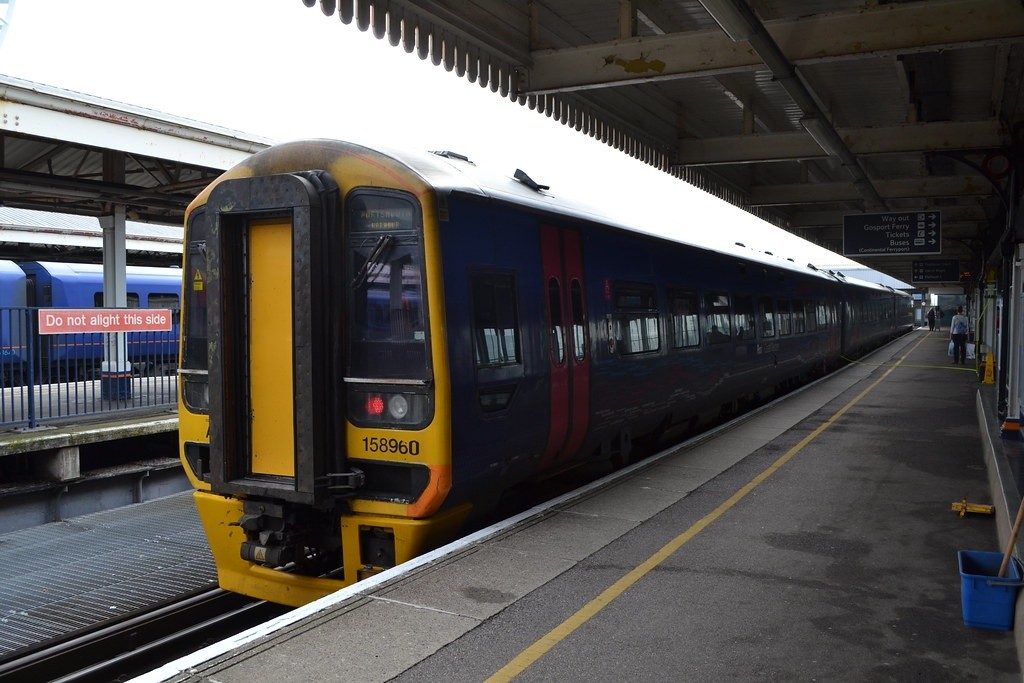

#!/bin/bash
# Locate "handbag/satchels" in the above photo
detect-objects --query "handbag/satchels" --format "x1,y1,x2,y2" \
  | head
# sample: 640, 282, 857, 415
966, 343, 975, 359
948, 340, 954, 356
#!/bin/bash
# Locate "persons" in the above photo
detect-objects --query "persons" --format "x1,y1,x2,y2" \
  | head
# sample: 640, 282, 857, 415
950, 306, 969, 365
709, 325, 729, 345
736, 320, 754, 340
927, 307, 943, 332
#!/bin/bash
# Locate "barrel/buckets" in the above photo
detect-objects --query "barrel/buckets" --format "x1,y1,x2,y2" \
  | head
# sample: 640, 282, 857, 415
958, 551, 1024, 631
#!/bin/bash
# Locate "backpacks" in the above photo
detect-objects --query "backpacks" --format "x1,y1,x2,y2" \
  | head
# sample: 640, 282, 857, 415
954, 315, 968, 337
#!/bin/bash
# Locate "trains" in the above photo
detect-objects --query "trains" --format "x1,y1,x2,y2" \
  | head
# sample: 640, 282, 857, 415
179, 137, 914, 607
0, 260, 182, 388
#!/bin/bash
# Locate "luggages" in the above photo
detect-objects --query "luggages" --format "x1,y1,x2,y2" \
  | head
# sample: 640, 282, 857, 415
935, 319, 940, 331
